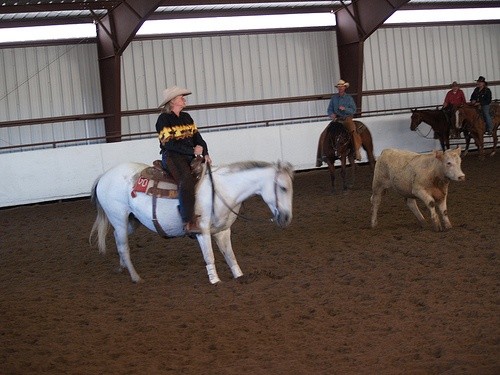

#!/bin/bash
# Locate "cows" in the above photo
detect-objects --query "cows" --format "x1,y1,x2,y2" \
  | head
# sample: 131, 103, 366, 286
367, 145, 467, 232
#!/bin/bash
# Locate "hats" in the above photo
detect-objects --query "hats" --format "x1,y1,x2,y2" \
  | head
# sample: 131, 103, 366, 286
474, 76, 487, 84
334, 80, 349, 88
158, 85, 192, 108
450, 82, 460, 88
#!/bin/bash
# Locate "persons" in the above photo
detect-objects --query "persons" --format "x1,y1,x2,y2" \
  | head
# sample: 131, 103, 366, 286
327, 80, 362, 163
469, 76, 494, 136
155, 85, 212, 233
442, 81, 467, 111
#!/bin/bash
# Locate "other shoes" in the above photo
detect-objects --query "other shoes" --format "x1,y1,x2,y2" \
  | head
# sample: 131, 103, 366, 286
487, 127, 492, 134
355, 153, 361, 161
316, 159, 322, 167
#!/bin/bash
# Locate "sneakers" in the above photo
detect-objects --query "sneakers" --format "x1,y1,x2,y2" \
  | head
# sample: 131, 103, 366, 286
184, 221, 201, 233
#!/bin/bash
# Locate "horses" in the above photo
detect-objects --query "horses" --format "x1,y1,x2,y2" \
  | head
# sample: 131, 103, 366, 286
409, 100, 500, 161
318, 117, 375, 192
87, 158, 294, 289
